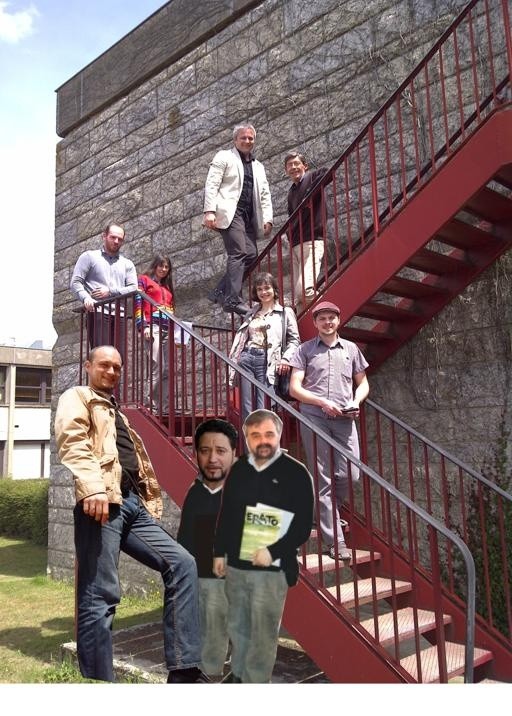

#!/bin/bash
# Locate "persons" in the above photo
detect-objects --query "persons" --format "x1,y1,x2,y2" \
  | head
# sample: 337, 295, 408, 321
279, 152, 333, 302
54, 344, 208, 682
289, 300, 370, 560
211, 408, 315, 684
226, 271, 301, 413
134, 252, 180, 413
171, 418, 238, 681
71, 222, 138, 361
200, 124, 273, 315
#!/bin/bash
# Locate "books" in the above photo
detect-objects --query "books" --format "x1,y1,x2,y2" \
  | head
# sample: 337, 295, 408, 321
233, 501, 295, 567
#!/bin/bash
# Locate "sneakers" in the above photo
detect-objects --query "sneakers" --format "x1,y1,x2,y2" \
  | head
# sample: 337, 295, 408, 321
166, 668, 215, 683
329, 542, 352, 560
339, 518, 349, 527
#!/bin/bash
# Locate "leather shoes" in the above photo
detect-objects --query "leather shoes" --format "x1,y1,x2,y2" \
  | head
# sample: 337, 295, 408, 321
208, 290, 224, 306
223, 302, 252, 314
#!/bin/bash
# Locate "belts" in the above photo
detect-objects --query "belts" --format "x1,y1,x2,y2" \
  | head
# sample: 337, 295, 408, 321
244, 346, 268, 356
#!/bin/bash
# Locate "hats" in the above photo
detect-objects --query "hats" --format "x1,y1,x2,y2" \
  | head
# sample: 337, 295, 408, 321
312, 302, 343, 317
